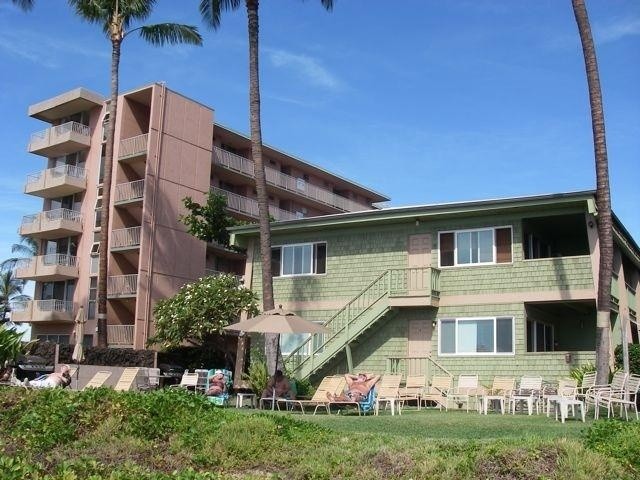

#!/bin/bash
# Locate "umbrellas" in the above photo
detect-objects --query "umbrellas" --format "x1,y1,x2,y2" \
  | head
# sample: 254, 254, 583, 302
71, 304, 88, 389
222, 303, 336, 411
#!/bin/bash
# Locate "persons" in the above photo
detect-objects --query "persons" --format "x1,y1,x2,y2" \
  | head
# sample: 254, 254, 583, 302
49, 365, 72, 387
326, 371, 382, 402
204, 370, 224, 397
260, 369, 294, 410
